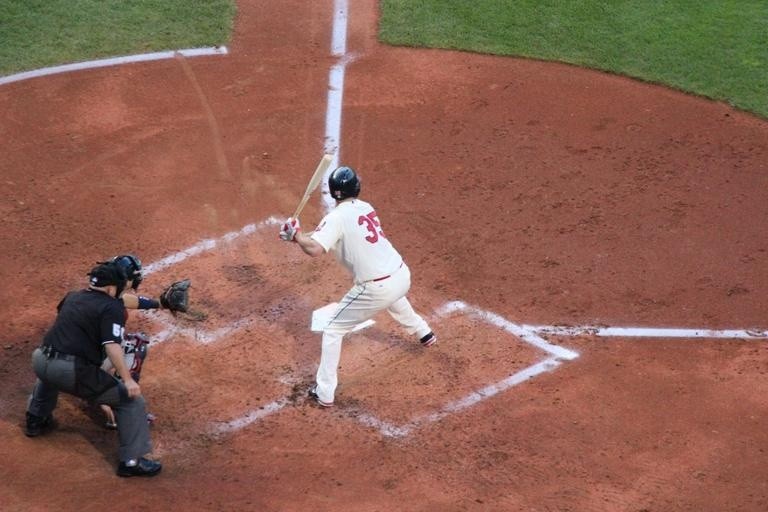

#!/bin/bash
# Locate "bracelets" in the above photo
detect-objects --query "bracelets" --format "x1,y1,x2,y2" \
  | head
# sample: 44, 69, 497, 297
135, 296, 152, 312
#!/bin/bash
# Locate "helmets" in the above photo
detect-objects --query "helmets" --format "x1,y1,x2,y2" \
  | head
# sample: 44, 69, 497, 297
329, 166, 361, 201
91, 255, 143, 299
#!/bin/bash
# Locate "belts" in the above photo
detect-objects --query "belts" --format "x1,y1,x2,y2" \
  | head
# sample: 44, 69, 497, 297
41, 345, 67, 360
373, 262, 404, 282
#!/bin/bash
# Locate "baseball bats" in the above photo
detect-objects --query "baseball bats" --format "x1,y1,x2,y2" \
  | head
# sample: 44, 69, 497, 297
291, 154, 333, 221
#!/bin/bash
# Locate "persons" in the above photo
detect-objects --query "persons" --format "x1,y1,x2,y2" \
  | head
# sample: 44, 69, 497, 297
96, 253, 191, 428
25, 260, 163, 478
278, 164, 437, 408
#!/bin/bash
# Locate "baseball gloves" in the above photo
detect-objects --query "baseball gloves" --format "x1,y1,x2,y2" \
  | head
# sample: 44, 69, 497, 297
163, 281, 191, 316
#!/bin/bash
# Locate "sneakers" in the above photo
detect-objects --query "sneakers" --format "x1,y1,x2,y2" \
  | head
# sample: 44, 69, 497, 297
420, 331, 436, 347
310, 384, 334, 407
118, 457, 161, 477
105, 420, 118, 430
25, 412, 52, 436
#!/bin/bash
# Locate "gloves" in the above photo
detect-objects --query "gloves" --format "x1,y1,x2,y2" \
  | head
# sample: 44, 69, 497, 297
279, 218, 303, 241
